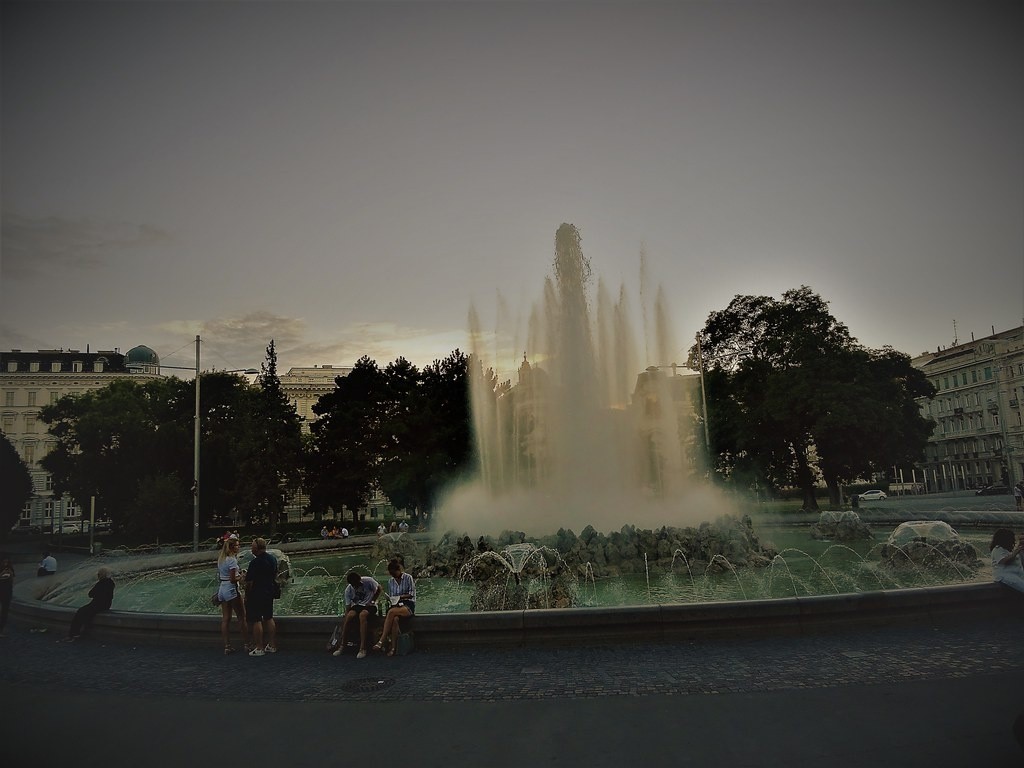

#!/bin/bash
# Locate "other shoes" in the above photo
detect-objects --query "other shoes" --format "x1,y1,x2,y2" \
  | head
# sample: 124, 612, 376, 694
357, 647, 369, 659
61, 632, 80, 643
386, 648, 397, 657
224, 646, 235, 655
332, 647, 347, 657
373, 641, 386, 652
244, 643, 253, 652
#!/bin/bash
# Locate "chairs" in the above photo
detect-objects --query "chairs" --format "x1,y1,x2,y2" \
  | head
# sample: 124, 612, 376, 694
99, 517, 447, 554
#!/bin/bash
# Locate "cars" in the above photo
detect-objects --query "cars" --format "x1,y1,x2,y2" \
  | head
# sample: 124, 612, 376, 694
857, 490, 888, 502
53, 523, 80, 535
63, 518, 115, 533
975, 483, 1011, 497
13, 524, 43, 535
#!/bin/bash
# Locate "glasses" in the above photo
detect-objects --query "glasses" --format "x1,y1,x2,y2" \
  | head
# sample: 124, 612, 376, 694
255, 538, 259, 548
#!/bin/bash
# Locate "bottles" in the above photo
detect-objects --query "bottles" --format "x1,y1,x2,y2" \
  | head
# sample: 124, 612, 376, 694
385, 601, 389, 616
378, 600, 382, 616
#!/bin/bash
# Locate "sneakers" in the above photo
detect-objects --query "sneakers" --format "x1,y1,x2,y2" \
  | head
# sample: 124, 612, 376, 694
264, 643, 277, 652
249, 648, 265, 656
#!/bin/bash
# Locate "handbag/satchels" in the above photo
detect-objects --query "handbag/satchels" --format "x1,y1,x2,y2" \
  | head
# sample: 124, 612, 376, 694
272, 583, 281, 599
212, 593, 221, 607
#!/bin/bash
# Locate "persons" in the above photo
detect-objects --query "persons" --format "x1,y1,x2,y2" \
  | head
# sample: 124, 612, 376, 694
37, 552, 56, 577
372, 560, 416, 656
991, 529, 1024, 592
217, 530, 239, 549
244, 538, 282, 656
975, 482, 979, 490
911, 484, 924, 495
333, 571, 382, 659
321, 526, 348, 540
418, 523, 425, 533
218, 537, 256, 654
1014, 481, 1024, 508
0, 557, 15, 638
62, 566, 115, 644
843, 492, 848, 504
377, 519, 409, 538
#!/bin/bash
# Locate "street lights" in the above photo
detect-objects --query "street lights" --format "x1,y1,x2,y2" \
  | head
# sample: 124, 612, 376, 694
125, 333, 259, 553
646, 348, 754, 488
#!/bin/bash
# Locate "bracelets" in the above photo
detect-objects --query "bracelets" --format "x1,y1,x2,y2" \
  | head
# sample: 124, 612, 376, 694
402, 595, 404, 599
239, 574, 242, 578
